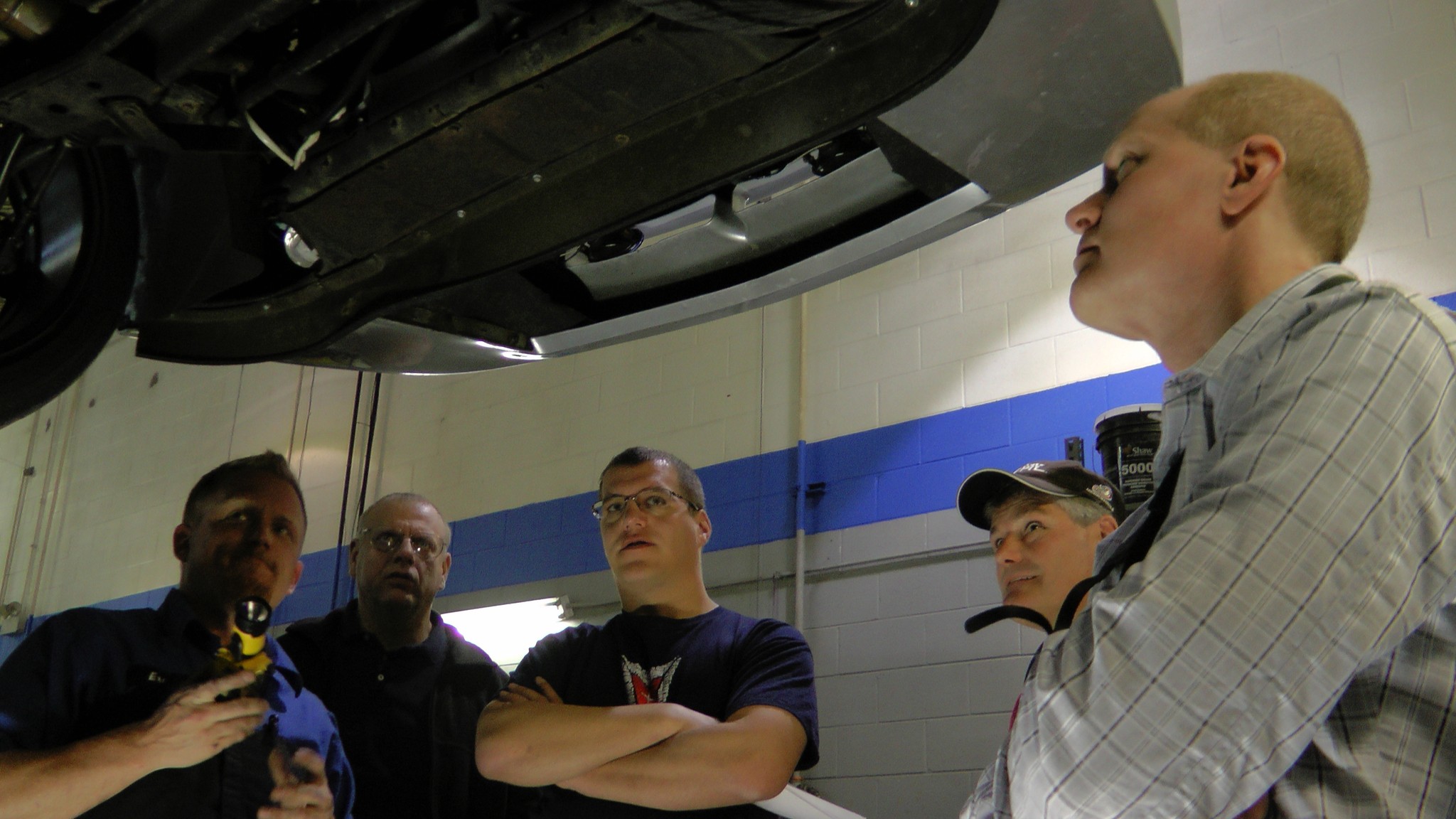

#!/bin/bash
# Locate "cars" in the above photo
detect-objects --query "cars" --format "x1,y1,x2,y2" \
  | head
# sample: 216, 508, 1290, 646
0, 0, 1185, 434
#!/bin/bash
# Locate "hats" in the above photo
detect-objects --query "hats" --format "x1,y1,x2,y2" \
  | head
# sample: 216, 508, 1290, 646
958, 460, 1125, 531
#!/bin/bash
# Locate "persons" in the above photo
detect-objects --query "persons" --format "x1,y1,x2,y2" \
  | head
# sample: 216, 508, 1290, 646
957, 459, 1125, 644
473, 447, 821, 819
0, 447, 360, 818
273, 494, 527, 818
996, 71, 1455, 819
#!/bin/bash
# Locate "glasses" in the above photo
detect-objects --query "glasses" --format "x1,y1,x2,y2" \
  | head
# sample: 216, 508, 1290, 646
358, 526, 448, 559
591, 486, 700, 524
